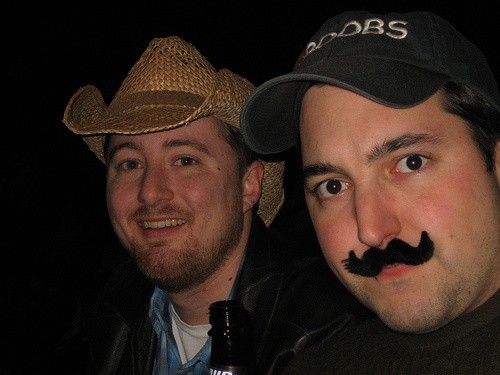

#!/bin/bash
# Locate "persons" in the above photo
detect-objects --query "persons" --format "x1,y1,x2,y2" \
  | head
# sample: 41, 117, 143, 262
239, 10, 500, 375
62, 35, 358, 375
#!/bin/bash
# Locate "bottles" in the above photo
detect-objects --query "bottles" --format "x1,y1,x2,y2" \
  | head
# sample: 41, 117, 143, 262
208, 300, 251, 375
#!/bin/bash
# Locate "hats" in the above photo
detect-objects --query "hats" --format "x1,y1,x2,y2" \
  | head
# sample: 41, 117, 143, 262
240, 12, 500, 155
61, 36, 284, 228
